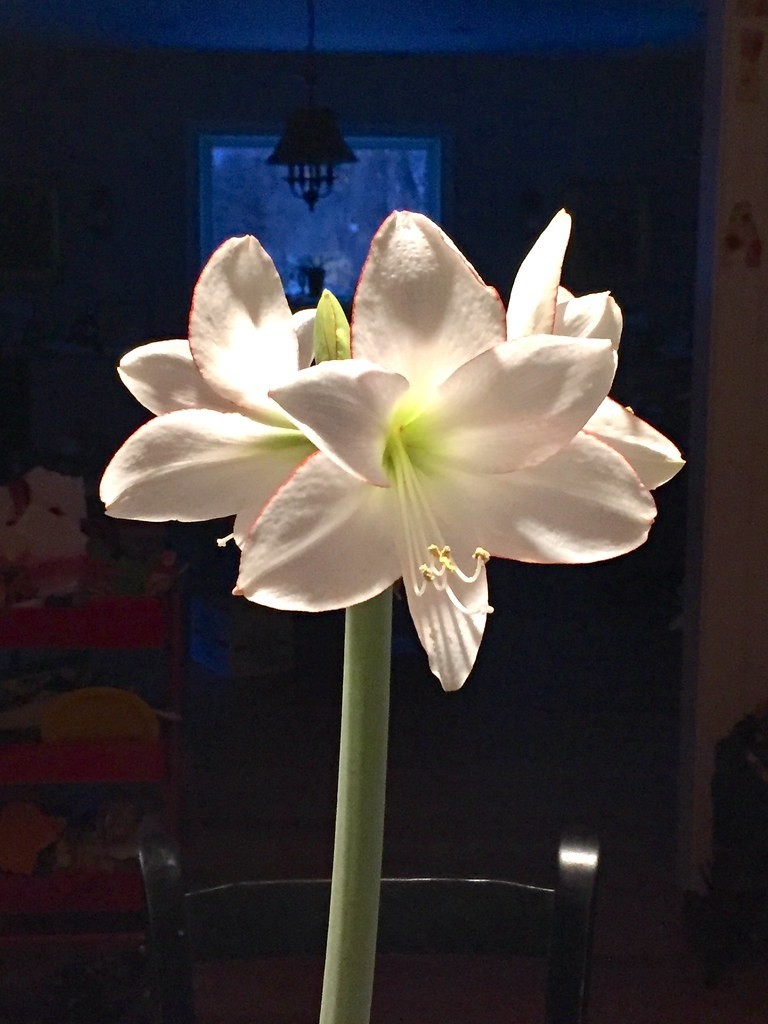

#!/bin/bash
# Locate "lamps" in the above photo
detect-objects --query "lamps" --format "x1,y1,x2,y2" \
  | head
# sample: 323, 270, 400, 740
260, 0, 363, 208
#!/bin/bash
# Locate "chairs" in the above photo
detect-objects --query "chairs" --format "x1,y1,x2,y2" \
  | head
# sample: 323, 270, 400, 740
136, 809, 604, 1024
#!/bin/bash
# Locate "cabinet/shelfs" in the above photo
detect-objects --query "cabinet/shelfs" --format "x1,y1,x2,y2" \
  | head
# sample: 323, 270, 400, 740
0, 537, 184, 949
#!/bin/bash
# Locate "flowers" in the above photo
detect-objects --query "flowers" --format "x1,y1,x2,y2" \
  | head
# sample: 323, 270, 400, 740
76, 210, 689, 1024
